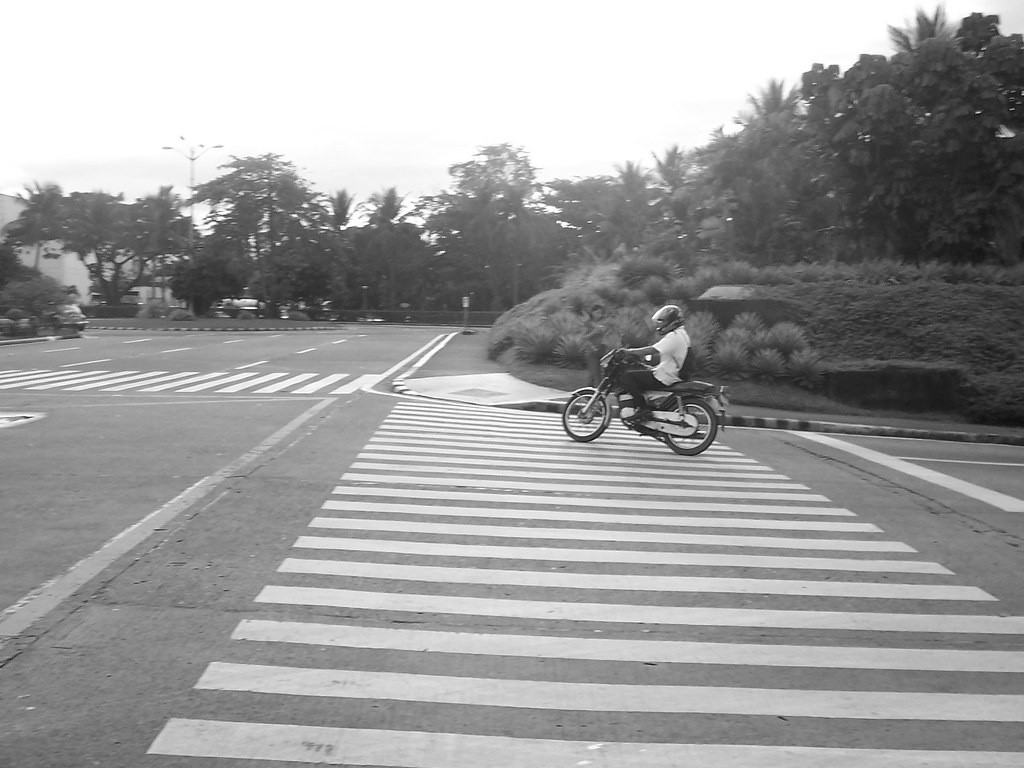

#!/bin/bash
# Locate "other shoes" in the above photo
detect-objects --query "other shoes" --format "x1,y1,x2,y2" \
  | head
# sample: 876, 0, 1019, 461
626, 408, 652, 421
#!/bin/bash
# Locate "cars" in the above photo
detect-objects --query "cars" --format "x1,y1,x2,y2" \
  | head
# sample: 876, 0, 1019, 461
238, 299, 259, 318
49, 302, 90, 330
148, 297, 171, 318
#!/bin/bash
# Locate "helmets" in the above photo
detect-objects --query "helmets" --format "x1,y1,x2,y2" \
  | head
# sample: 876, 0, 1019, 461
651, 304, 684, 335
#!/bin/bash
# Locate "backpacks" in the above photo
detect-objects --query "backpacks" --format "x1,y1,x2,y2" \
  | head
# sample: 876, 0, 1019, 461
669, 331, 697, 382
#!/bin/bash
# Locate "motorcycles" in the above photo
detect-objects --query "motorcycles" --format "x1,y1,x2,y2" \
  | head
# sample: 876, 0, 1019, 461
563, 334, 730, 457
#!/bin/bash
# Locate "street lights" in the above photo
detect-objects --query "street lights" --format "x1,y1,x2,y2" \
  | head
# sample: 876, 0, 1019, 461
162, 136, 224, 319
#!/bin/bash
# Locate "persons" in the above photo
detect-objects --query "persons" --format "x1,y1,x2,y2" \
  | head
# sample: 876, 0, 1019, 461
616, 305, 693, 422
